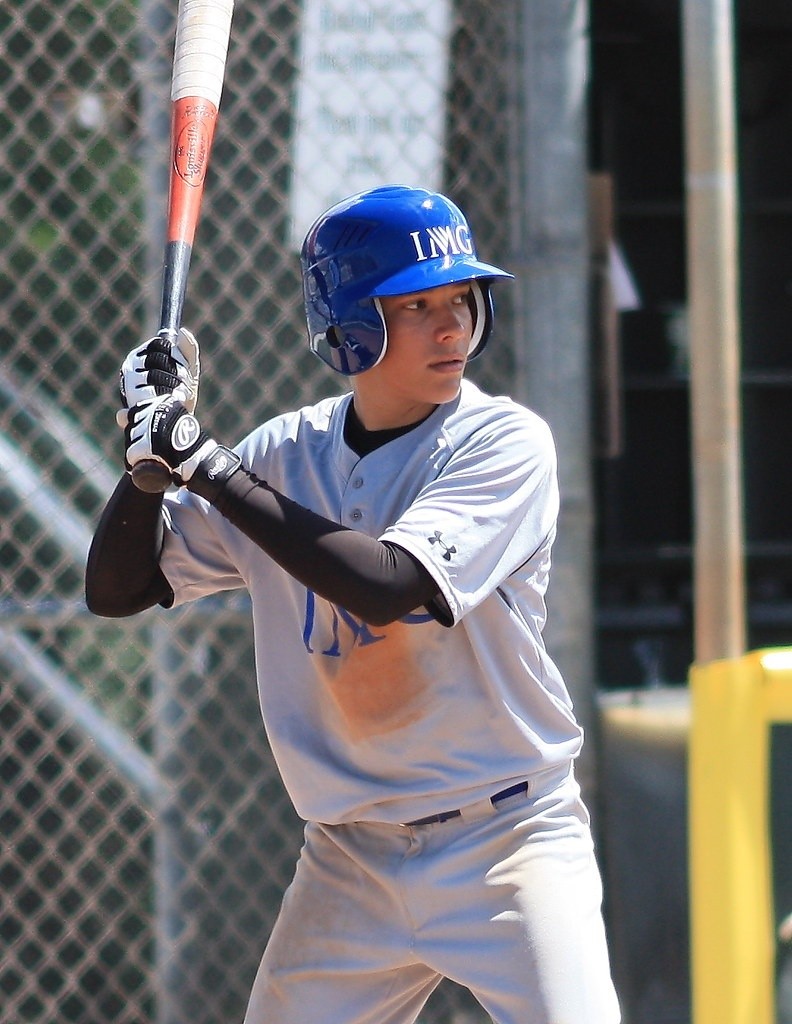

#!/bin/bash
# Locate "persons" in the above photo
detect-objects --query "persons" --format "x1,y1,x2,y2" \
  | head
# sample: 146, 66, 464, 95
85, 185, 622, 1024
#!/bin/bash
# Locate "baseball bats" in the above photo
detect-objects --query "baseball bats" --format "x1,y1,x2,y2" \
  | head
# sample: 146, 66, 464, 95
127, 0, 238, 492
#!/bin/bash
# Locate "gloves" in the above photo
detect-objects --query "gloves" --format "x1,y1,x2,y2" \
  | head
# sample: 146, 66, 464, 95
119, 328, 200, 416
115, 394, 243, 504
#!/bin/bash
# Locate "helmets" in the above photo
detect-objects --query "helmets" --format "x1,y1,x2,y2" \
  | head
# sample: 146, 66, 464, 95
300, 184, 516, 375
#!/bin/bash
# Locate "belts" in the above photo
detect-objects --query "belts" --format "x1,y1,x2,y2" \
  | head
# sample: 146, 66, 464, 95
398, 780, 528, 830
